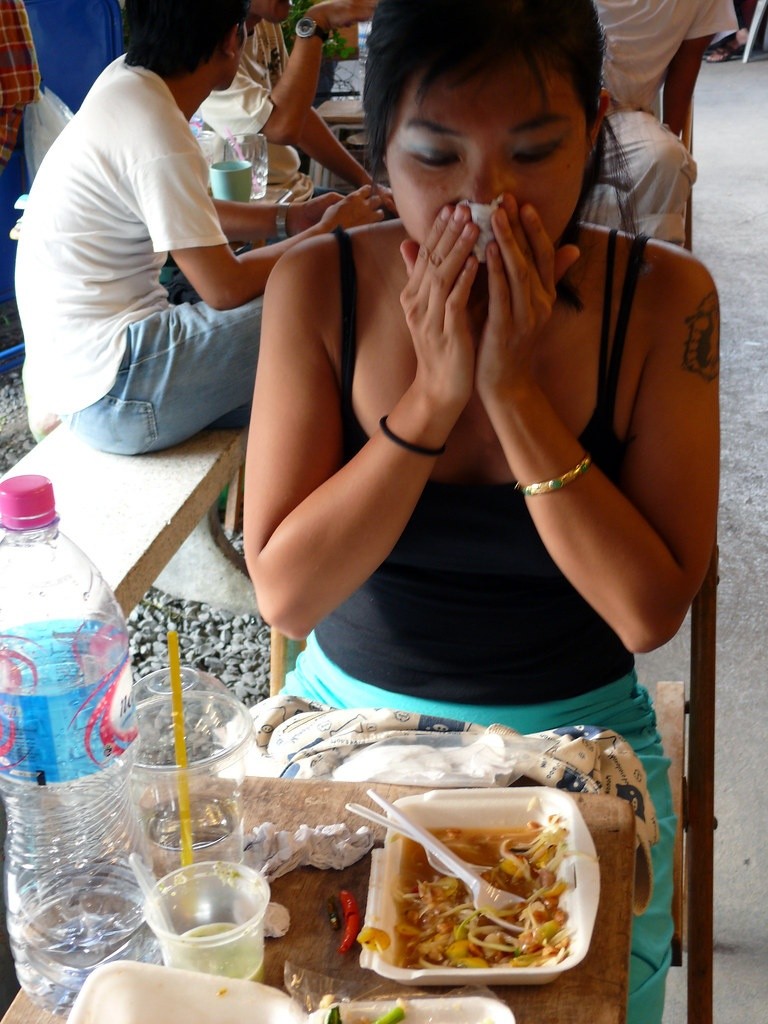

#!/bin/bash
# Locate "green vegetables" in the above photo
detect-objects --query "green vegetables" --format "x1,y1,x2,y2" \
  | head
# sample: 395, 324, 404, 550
325, 1005, 405, 1024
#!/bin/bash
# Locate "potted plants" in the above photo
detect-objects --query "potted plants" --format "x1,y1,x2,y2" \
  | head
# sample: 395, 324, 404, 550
282, 0, 354, 101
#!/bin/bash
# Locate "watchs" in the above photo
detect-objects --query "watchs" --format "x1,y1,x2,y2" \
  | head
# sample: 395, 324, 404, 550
294, 16, 328, 44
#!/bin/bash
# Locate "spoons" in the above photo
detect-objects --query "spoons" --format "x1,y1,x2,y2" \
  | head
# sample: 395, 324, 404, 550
367, 788, 527, 932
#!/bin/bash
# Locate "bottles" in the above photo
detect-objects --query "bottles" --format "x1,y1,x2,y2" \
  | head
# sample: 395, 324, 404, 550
0, 469, 163, 1016
189, 106, 202, 142
358, 22, 371, 65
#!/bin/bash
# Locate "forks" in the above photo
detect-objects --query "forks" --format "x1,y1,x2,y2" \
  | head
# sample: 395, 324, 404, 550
345, 801, 492, 879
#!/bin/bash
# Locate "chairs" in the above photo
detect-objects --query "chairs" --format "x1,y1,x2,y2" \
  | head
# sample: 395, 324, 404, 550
269, 540, 720, 1024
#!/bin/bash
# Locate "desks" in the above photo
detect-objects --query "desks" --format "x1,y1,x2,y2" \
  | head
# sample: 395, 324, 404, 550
0, 773, 636, 1024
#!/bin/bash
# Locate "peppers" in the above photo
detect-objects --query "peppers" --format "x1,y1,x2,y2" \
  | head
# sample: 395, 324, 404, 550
338, 889, 361, 955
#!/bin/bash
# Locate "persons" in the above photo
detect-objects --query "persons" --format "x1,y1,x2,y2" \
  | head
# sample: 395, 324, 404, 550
13, 0, 398, 457
576, 0, 720, 250
238, 0, 725, 1023
704, 0, 754, 63
186, 0, 396, 219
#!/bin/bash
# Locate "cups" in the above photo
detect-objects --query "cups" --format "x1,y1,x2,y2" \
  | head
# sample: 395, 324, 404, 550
197, 131, 225, 196
129, 667, 255, 884
143, 861, 270, 984
225, 133, 268, 199
209, 160, 253, 203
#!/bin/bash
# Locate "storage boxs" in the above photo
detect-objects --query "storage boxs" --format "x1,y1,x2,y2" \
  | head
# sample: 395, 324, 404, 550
67, 960, 517, 1024
357, 784, 602, 986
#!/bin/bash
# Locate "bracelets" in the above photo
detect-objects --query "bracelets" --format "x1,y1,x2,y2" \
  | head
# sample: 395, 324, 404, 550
274, 202, 290, 239
377, 411, 449, 460
513, 448, 594, 495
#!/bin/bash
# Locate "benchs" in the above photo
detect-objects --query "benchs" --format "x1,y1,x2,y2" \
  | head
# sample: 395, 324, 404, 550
0, 405, 252, 628
